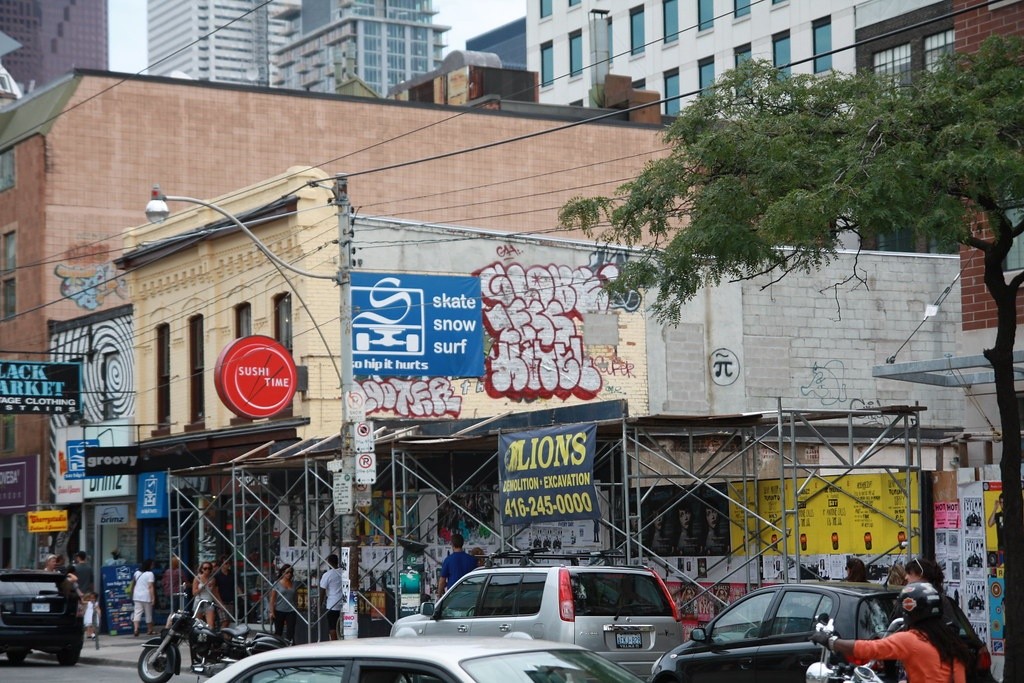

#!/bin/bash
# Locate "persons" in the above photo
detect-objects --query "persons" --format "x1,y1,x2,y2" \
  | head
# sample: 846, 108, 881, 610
668, 582, 741, 614
42, 550, 343, 642
967, 493, 1005, 610
809, 558, 968, 683
438, 534, 477, 599
533, 500, 722, 557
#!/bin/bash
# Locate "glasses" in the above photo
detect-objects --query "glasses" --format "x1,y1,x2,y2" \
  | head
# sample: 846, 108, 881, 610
202, 567, 212, 570
912, 557, 923, 575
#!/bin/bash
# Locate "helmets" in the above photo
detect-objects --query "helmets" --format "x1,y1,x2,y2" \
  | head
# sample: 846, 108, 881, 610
897, 582, 944, 629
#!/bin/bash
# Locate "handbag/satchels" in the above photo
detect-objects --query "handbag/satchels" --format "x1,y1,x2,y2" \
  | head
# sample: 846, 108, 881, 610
124, 578, 135, 597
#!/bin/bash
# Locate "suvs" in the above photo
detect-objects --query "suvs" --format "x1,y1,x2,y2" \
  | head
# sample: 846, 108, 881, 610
0, 568, 85, 666
390, 547, 684, 683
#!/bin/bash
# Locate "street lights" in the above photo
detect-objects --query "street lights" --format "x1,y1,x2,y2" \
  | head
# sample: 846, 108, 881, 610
145, 184, 358, 637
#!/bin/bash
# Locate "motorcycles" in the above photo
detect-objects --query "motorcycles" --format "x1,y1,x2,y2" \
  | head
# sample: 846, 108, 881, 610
804, 613, 907, 683
137, 582, 289, 683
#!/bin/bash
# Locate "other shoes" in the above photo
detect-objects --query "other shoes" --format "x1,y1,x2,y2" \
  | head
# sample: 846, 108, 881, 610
135, 632, 138, 636
147, 631, 152, 634
92, 635, 96, 640
87, 635, 91, 639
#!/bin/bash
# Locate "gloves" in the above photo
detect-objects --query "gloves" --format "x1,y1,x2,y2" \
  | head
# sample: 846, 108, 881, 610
808, 620, 834, 651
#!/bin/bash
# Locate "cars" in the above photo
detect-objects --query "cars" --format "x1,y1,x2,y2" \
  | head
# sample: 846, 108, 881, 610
203, 638, 644, 683
647, 581, 902, 683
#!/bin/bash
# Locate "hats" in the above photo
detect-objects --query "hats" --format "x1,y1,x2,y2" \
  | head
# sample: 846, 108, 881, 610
110, 547, 120, 555
46, 553, 57, 562
